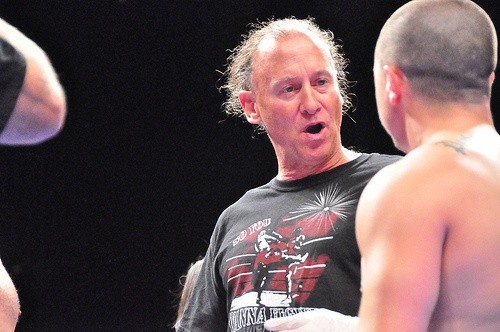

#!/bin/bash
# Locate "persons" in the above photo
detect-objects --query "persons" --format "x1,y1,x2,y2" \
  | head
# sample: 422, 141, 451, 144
354, 0, 500, 331
0, 15, 66, 332
172, 14, 407, 332
